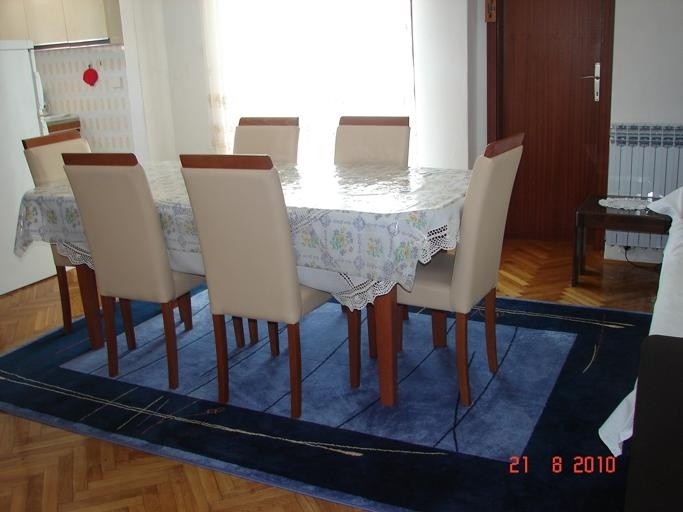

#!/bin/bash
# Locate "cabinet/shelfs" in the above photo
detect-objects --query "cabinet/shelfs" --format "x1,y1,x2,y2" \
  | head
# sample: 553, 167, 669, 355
24, 0, 124, 51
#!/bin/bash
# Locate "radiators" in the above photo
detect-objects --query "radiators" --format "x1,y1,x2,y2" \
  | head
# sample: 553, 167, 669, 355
606, 125, 683, 249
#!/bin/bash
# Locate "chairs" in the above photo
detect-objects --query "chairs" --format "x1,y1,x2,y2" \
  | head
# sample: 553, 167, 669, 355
334, 116, 410, 354
235, 117, 300, 355
61, 152, 246, 390
348, 130, 526, 405
22, 128, 192, 350
179, 154, 361, 417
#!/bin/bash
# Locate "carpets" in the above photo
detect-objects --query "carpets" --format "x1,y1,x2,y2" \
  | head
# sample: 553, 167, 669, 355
0, 283, 652, 512
